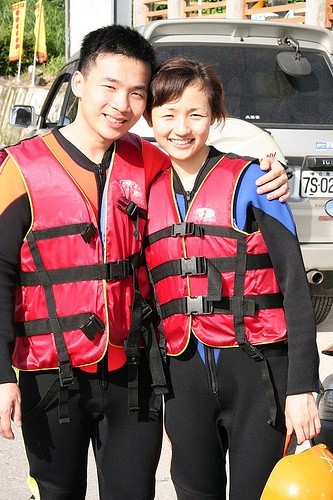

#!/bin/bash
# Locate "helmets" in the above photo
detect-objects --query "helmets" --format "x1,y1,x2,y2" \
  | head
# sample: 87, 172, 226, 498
260, 428, 333, 500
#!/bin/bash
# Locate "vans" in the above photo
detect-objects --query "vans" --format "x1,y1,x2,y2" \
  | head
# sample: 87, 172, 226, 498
8, 18, 333, 326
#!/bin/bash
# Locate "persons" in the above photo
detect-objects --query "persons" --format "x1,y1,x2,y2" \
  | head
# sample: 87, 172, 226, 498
144, 56, 322, 500
0, 24, 290, 500
